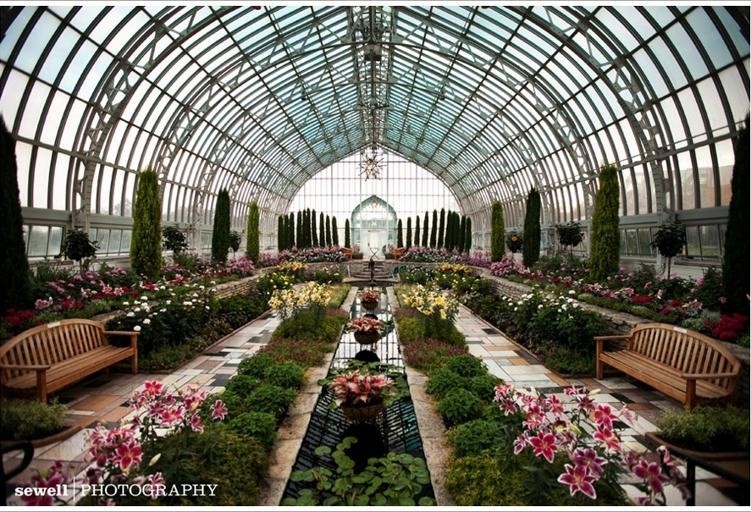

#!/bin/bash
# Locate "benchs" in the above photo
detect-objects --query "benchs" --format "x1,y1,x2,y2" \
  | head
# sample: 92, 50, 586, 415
0, 318, 140, 408
589, 321, 744, 413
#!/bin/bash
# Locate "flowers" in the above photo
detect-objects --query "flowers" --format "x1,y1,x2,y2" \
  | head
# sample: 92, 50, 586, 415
328, 281, 391, 408
18, 378, 228, 505
503, 382, 685, 504
27, 240, 361, 330
385, 240, 739, 333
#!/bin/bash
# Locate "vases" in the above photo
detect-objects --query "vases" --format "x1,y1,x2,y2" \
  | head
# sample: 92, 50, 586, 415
338, 402, 383, 422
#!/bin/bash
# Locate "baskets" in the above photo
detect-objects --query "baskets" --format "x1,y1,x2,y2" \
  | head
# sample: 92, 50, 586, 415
363, 303, 378, 310
341, 398, 384, 418
354, 333, 379, 344
